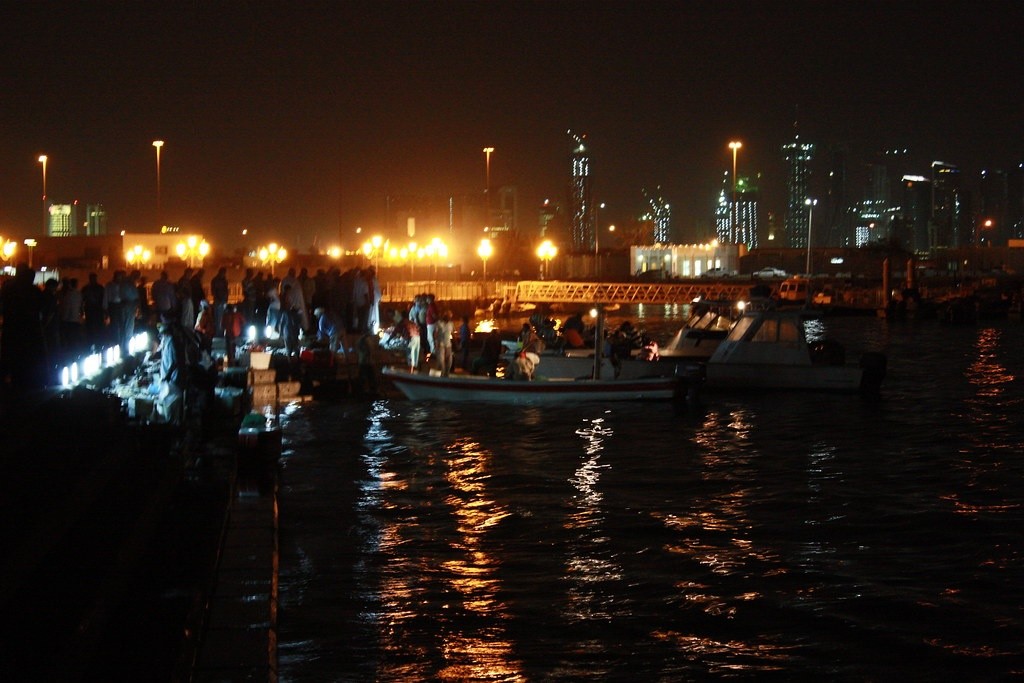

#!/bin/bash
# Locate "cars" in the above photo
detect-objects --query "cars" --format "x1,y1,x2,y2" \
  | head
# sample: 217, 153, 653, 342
635, 269, 671, 285
700, 267, 731, 280
752, 266, 787, 280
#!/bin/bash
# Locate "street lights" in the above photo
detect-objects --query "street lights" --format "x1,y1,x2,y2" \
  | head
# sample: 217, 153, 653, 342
129, 245, 152, 272
535, 240, 558, 281
424, 237, 448, 281
729, 142, 742, 245
976, 219, 993, 247
476, 237, 495, 282
175, 235, 209, 268
483, 147, 494, 237
804, 198, 817, 275
39, 155, 48, 237
260, 242, 288, 280
401, 240, 424, 281
152, 141, 165, 210
363, 235, 390, 278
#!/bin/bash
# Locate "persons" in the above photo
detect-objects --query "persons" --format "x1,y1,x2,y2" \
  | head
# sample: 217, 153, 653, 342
586, 320, 651, 382
470, 330, 502, 375
461, 315, 471, 370
0, 261, 378, 432
381, 294, 457, 378
517, 311, 585, 351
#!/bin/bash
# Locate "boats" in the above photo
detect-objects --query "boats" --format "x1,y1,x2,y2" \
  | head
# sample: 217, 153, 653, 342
380, 255, 1024, 417
